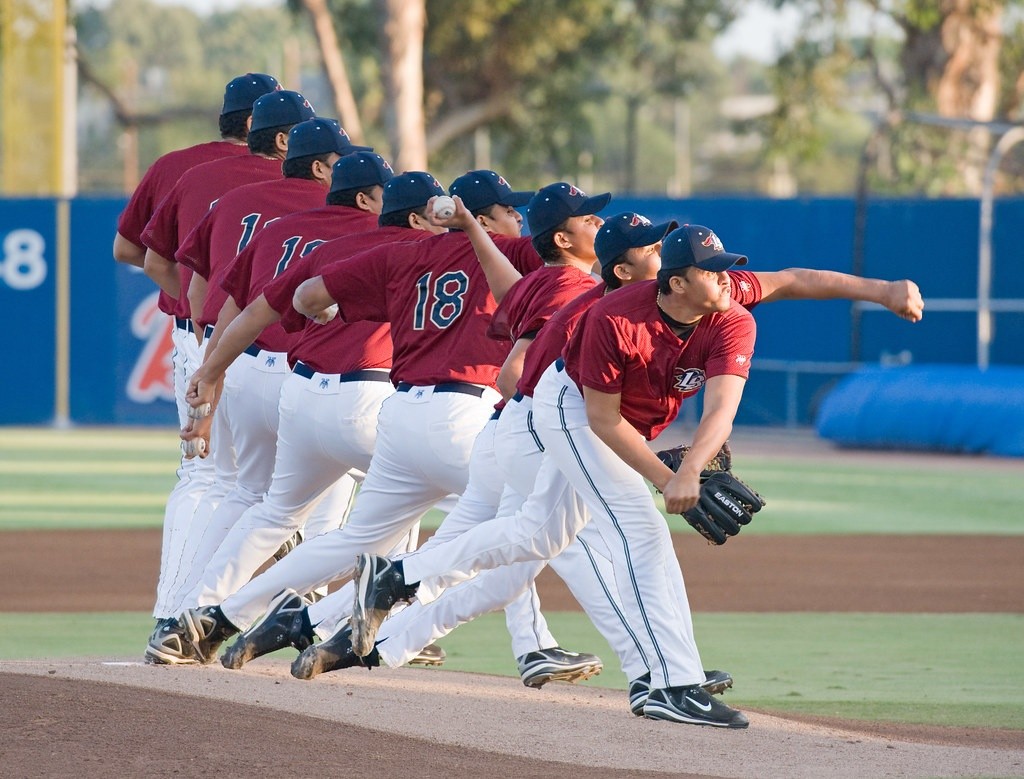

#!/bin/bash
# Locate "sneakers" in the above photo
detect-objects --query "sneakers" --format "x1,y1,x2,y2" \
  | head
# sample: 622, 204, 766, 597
143, 619, 196, 664
178, 606, 231, 663
643, 685, 749, 729
410, 643, 447, 667
290, 616, 380, 680
517, 646, 603, 690
629, 670, 733, 714
351, 553, 399, 656
220, 587, 313, 668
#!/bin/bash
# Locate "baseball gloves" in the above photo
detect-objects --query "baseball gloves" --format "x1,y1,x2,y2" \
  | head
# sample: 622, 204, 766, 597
653, 440, 733, 494
678, 469, 766, 547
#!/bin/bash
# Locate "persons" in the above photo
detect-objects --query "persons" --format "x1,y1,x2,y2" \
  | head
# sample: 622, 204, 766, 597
113, 73, 926, 730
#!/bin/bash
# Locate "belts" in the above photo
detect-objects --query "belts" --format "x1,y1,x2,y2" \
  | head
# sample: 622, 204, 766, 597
176, 316, 194, 332
204, 327, 214, 338
491, 409, 501, 420
398, 383, 484, 397
293, 363, 390, 383
512, 391, 525, 402
244, 344, 261, 357
555, 356, 566, 372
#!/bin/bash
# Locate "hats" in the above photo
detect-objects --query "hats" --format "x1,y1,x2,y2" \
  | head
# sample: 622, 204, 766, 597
527, 182, 611, 241
448, 170, 535, 211
594, 212, 679, 268
382, 172, 446, 215
285, 117, 374, 160
220, 72, 285, 114
661, 224, 748, 272
330, 151, 393, 193
250, 89, 316, 131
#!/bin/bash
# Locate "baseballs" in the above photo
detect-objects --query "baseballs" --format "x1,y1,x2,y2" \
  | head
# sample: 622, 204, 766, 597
432, 195, 457, 219
186, 402, 211, 418
180, 437, 206, 456
